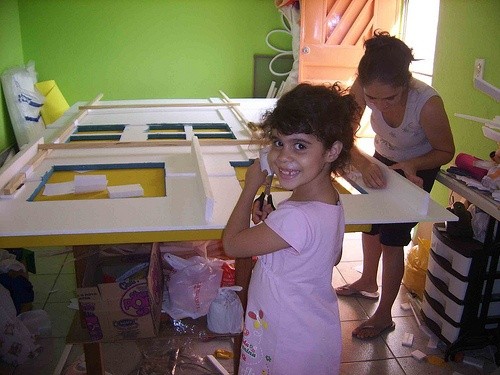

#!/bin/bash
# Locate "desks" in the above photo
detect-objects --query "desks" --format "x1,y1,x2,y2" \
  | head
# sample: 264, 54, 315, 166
436, 169, 500, 367
0, 98, 460, 375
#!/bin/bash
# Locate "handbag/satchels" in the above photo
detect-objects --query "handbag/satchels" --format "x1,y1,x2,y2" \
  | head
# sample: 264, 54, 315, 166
207, 286, 244, 334
163, 253, 223, 318
1, 61, 44, 150
0, 284, 50, 364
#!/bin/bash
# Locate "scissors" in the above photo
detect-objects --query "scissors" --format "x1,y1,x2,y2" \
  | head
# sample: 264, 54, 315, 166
251, 173, 276, 224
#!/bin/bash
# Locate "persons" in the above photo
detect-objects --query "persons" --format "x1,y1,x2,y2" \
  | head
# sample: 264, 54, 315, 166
221, 83, 360, 374
334, 29, 455, 339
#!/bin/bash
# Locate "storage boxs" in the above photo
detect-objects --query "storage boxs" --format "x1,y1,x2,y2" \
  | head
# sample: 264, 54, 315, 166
75, 243, 164, 344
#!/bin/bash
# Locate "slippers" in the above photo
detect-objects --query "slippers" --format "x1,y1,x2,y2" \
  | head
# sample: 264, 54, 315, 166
352, 321, 395, 339
335, 284, 379, 299
